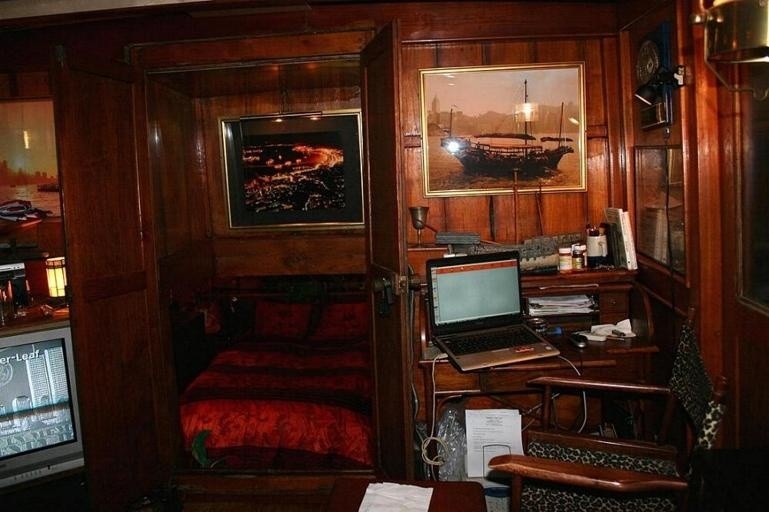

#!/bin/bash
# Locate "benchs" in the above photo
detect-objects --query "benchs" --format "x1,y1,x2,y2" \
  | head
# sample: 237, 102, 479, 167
177, 446, 376, 512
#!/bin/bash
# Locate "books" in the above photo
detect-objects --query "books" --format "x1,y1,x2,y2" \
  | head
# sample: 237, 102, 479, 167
465, 408, 525, 477
528, 295, 594, 316
603, 207, 638, 271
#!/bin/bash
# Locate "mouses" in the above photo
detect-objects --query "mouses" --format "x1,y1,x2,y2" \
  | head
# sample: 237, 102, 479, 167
570, 334, 588, 348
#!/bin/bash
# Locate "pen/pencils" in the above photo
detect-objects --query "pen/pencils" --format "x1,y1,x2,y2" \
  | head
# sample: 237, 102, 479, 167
607, 337, 624, 341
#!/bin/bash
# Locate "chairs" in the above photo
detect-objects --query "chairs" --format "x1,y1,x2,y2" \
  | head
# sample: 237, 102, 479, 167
488, 304, 730, 512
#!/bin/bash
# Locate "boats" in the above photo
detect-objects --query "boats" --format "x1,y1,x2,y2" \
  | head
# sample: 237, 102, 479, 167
436, 77, 577, 180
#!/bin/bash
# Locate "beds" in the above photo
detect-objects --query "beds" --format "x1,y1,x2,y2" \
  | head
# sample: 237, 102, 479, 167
176, 273, 378, 467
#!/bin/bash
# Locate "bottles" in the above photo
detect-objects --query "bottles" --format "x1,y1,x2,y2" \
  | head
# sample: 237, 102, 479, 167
558, 244, 588, 273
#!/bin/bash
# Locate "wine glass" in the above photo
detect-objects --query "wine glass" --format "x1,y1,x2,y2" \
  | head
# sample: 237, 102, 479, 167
408, 207, 429, 248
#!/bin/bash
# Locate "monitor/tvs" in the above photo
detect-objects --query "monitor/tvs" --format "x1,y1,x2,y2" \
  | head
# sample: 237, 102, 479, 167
0, 327, 85, 488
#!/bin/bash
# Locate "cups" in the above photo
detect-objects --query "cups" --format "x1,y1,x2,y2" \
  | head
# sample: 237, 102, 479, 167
586, 235, 608, 268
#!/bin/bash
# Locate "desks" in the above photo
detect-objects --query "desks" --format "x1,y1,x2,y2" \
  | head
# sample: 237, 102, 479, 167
408, 241, 662, 481
326, 477, 488, 512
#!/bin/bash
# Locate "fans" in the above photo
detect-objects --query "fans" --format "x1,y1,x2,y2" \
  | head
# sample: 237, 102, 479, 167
636, 38, 686, 89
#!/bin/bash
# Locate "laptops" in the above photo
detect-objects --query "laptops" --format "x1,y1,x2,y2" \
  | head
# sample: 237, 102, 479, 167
425, 252, 560, 372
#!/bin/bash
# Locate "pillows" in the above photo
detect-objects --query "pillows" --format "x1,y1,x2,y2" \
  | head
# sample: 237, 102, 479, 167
254, 299, 314, 340
308, 300, 370, 343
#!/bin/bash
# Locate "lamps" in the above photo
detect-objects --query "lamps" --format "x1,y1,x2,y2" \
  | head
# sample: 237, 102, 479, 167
46, 256, 71, 308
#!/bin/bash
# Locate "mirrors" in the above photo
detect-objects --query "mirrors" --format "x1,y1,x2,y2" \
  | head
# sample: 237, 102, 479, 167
634, 144, 688, 279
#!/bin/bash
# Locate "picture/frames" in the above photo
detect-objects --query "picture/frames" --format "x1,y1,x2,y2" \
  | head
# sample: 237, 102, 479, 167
636, 21, 673, 132
419, 61, 589, 199
217, 108, 368, 234
